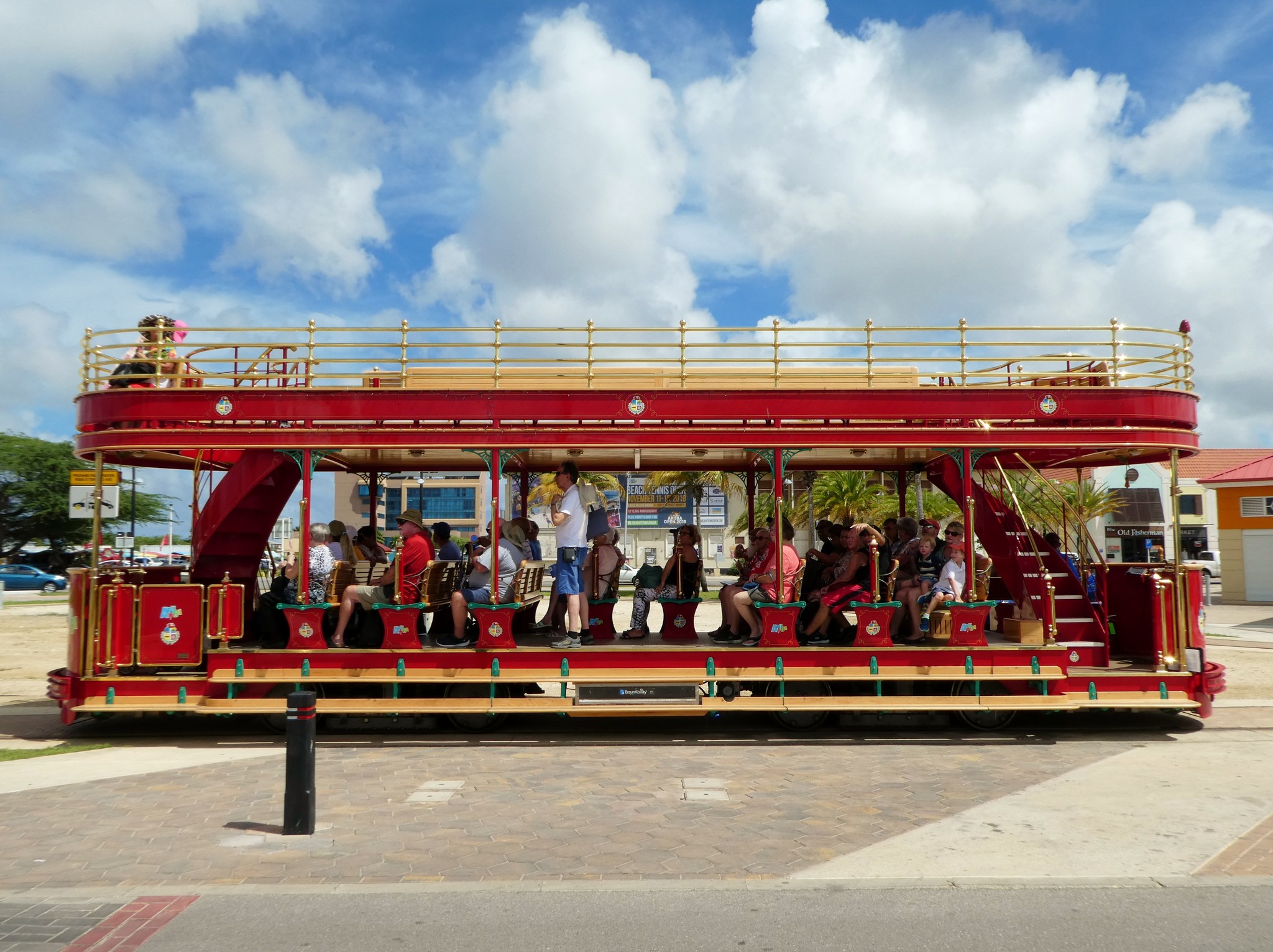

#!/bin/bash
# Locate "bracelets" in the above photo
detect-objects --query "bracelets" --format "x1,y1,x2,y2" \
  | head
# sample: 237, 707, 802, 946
287, 554, 296, 559
551, 504, 556, 506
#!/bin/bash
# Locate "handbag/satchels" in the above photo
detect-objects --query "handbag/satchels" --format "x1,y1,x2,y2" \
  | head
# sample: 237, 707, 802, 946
807, 589, 821, 603
742, 582, 761, 591
586, 504, 611, 542
270, 567, 291, 599
109, 362, 155, 386
563, 548, 577, 562
633, 563, 663, 588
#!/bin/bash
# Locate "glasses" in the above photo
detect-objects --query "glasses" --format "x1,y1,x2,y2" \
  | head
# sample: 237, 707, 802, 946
921, 525, 936, 529
944, 529, 962, 537
397, 519, 409, 525
556, 472, 564, 476
149, 323, 158, 327
748, 528, 775, 541
673, 531, 692, 537
861, 534, 871, 536
486, 527, 491, 533
884, 524, 898, 531
815, 525, 830, 530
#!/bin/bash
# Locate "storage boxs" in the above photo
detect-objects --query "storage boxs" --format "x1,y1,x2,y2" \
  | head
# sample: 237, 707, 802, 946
1002, 616, 1044, 645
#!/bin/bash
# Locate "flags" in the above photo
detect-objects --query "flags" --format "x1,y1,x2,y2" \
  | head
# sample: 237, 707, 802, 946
160, 534, 169, 551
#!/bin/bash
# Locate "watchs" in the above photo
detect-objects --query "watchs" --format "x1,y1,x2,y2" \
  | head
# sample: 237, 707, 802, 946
379, 577, 381, 581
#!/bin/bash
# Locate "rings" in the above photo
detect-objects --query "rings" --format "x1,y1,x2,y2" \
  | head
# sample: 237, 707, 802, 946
659, 588, 660, 590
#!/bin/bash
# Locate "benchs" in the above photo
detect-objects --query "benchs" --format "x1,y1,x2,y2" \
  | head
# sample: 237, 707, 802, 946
275, 558, 392, 651
845, 563, 922, 647
371, 558, 469, 649
586, 557, 624, 641
922, 558, 999, 646
1029, 360, 1111, 386
658, 559, 703, 640
469, 560, 548, 645
720, 557, 808, 647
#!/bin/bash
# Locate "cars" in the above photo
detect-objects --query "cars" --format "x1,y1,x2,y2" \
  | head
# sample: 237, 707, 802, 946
98, 557, 192, 573
618, 563, 644, 587
0, 564, 68, 593
259, 550, 283, 571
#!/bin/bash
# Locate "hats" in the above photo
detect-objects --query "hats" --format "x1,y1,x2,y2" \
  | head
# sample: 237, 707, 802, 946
918, 519, 940, 530
327, 520, 359, 541
948, 540, 965, 552
579, 478, 598, 514
766, 516, 787, 523
861, 525, 881, 535
669, 528, 680, 534
395, 509, 525, 548
139, 316, 174, 341
748, 527, 766, 535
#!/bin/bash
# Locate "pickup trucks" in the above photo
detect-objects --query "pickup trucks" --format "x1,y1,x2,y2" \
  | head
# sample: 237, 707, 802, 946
1182, 550, 1223, 585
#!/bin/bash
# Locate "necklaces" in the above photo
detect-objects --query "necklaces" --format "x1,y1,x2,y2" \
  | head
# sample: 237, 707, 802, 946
603, 543, 612, 545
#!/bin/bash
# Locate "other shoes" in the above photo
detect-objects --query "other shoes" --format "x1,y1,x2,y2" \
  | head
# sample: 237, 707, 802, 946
265, 590, 936, 648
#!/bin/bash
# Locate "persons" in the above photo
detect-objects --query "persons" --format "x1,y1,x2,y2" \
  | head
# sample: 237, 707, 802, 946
646, 526, 680, 634
708, 512, 989, 645
545, 514, 555, 528
1046, 533, 1080, 585
1182, 548, 1188, 559
917, 540, 966, 632
1079, 558, 1096, 607
104, 314, 188, 389
619, 522, 702, 639
325, 509, 542, 648
531, 460, 622, 648
899, 536, 944, 595
512, 494, 522, 519
251, 520, 387, 648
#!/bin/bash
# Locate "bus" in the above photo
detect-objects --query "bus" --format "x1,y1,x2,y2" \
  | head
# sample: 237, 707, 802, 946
47, 319, 1227, 727
